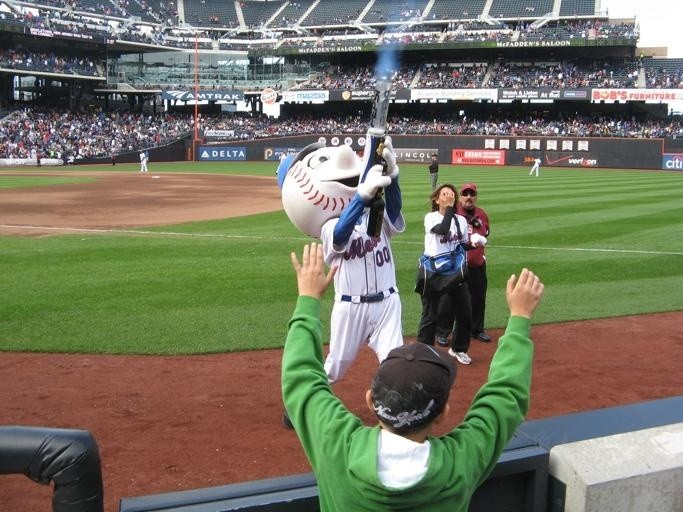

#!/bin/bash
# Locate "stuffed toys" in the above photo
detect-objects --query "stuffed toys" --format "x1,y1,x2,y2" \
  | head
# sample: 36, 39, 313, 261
276, 134, 404, 429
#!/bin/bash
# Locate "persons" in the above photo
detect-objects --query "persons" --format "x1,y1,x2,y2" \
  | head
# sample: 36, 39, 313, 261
427, 152, 438, 191
280, 241, 546, 512
432, 181, 490, 346
0, 1, 682, 172
528, 157, 541, 176
415, 182, 485, 366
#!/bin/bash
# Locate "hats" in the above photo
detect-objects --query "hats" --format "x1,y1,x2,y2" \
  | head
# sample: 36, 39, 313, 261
461, 184, 476, 192
371, 341, 457, 428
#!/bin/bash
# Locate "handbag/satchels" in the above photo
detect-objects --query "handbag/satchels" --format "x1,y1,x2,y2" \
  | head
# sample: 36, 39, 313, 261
414, 244, 466, 298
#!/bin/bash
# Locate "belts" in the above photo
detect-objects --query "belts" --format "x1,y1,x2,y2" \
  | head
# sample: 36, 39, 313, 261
341, 288, 395, 301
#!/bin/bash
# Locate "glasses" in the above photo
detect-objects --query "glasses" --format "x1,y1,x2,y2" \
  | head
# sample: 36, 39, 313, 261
463, 192, 475, 196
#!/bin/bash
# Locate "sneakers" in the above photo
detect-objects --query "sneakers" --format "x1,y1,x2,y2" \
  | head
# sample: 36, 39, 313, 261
474, 333, 489, 341
448, 348, 471, 364
437, 336, 449, 347
